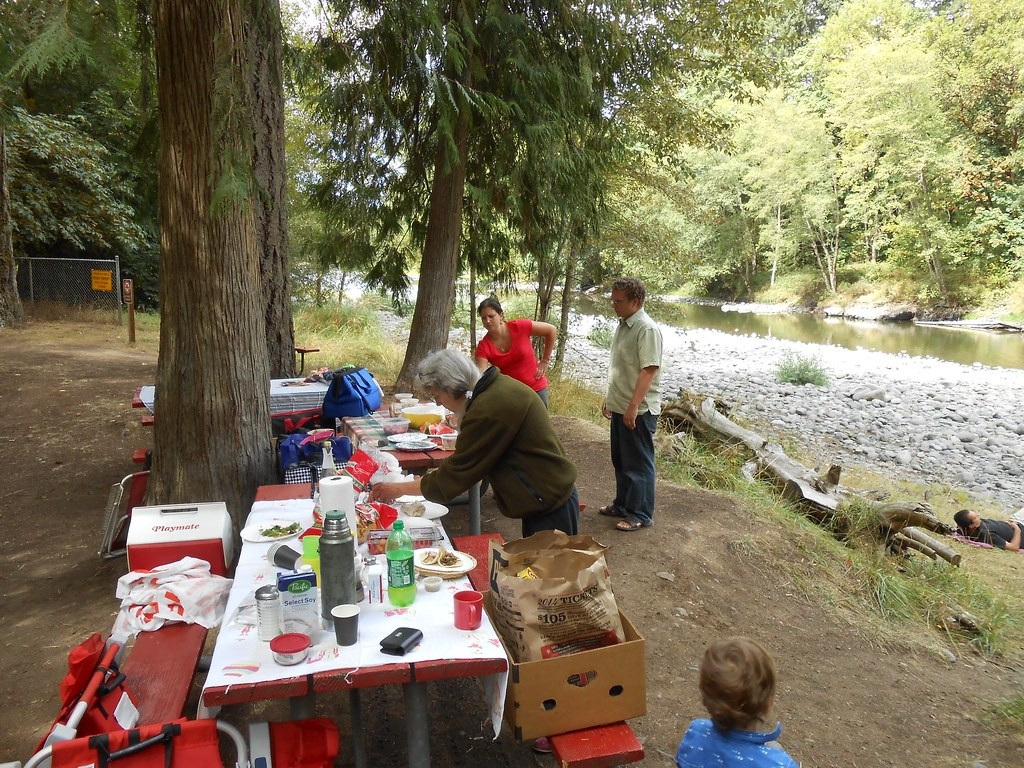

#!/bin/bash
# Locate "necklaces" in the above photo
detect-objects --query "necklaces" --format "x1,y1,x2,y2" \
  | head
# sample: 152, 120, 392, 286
488, 322, 506, 352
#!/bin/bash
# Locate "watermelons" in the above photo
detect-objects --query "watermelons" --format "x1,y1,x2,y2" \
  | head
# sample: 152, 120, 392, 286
419, 414, 460, 445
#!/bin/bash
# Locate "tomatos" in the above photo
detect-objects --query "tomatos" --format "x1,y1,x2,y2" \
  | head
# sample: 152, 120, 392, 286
368, 534, 433, 555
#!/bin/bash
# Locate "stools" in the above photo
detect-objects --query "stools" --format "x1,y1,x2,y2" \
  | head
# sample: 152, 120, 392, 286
294, 346, 320, 375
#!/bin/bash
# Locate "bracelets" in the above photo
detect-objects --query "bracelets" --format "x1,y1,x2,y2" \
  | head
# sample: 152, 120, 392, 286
541, 359, 549, 365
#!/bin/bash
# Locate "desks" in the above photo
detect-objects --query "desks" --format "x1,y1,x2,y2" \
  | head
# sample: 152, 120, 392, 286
132, 377, 332, 414
195, 482, 510, 768
344, 400, 481, 537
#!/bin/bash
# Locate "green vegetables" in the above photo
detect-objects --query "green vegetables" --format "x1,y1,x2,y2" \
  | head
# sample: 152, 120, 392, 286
259, 522, 301, 537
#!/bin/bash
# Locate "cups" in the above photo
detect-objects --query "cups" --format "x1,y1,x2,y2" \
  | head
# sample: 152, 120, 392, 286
267, 543, 304, 570
454, 591, 483, 630
303, 534, 321, 588
331, 604, 361, 646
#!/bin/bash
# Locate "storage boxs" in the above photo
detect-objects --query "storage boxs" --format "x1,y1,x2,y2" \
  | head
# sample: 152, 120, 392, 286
127, 501, 235, 574
478, 589, 647, 741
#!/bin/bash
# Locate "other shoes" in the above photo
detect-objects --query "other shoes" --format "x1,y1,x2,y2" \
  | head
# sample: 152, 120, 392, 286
532, 737, 553, 752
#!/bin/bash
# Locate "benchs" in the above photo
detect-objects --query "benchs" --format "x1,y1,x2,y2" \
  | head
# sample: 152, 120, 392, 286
116, 622, 208, 767
453, 532, 646, 768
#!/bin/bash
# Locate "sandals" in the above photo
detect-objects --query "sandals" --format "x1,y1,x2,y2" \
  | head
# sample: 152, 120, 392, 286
616, 517, 643, 531
599, 505, 627, 518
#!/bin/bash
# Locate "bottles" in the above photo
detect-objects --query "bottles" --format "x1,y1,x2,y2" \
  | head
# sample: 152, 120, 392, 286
319, 510, 356, 632
385, 520, 417, 607
354, 550, 365, 603
320, 441, 338, 479
362, 556, 385, 604
255, 585, 283, 641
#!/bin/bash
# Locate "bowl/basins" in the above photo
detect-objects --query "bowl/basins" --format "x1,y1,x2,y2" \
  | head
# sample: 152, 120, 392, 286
401, 407, 445, 429
400, 399, 419, 408
441, 434, 457, 448
395, 394, 413, 403
423, 576, 443, 592
382, 419, 411, 435
426, 430, 458, 447
270, 634, 310, 665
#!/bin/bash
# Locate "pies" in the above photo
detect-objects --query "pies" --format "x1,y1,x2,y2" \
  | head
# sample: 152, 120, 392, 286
401, 501, 426, 517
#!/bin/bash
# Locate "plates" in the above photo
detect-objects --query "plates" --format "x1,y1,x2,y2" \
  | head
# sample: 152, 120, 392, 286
387, 433, 427, 442
399, 517, 435, 529
396, 442, 437, 450
240, 522, 302, 543
393, 501, 449, 519
414, 548, 478, 577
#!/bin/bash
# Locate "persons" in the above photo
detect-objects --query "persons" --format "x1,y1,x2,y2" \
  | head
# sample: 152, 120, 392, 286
475, 294, 556, 409
675, 636, 801, 768
367, 349, 579, 538
598, 277, 663, 533
954, 507, 1024, 552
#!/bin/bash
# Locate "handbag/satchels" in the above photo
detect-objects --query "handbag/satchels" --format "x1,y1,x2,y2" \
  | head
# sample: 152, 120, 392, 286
276, 427, 353, 485
321, 366, 382, 417
